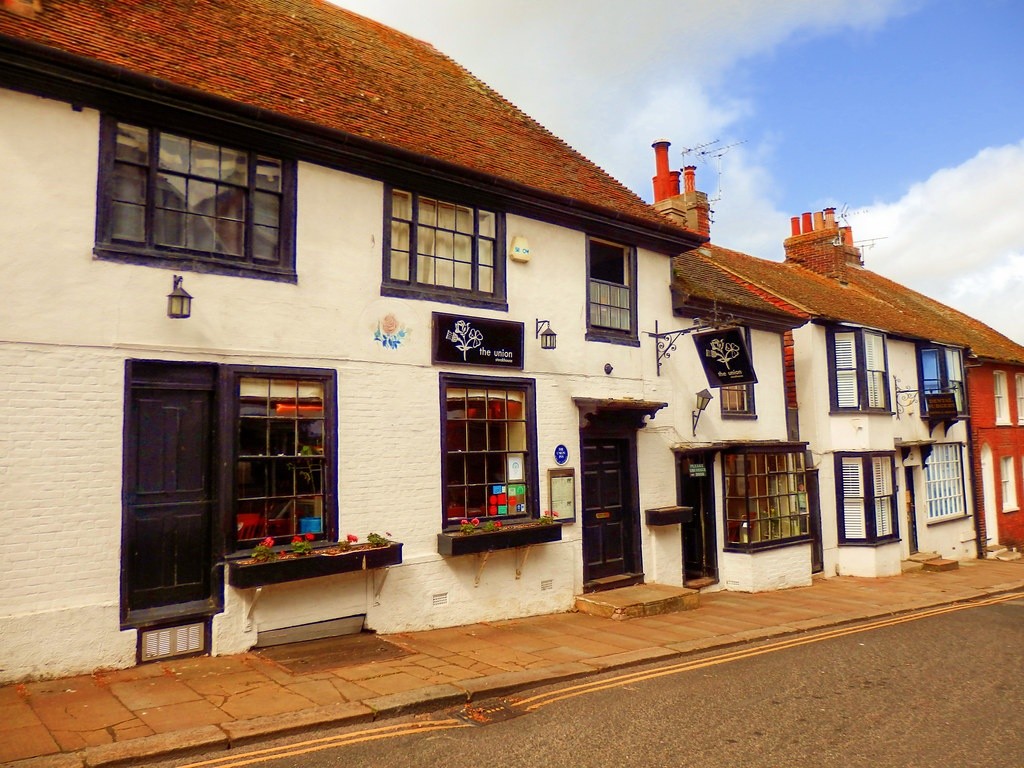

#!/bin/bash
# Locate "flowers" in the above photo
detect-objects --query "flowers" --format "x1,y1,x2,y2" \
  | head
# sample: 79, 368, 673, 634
460, 511, 559, 536
251, 532, 391, 563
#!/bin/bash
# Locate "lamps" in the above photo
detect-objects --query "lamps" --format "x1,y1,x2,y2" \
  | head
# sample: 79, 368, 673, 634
691, 389, 713, 437
535, 318, 556, 349
166, 274, 192, 319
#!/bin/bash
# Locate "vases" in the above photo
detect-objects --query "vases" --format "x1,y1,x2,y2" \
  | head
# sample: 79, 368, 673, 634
437, 522, 562, 556
228, 540, 403, 590
